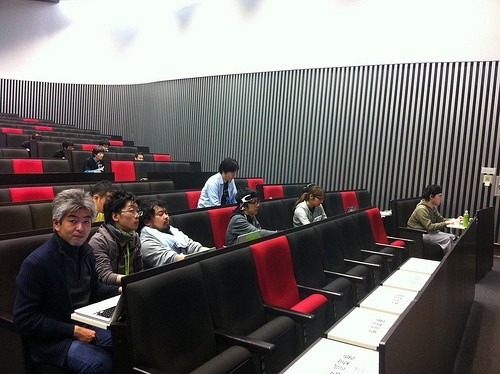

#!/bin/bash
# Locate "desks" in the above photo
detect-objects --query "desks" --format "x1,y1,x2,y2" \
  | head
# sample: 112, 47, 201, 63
277, 206, 496, 374
70, 294, 122, 330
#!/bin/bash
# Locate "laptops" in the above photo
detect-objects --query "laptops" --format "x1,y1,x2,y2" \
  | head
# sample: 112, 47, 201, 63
71, 291, 124, 326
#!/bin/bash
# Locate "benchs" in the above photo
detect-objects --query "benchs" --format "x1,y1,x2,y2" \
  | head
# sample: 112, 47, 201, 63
0, 112, 445, 374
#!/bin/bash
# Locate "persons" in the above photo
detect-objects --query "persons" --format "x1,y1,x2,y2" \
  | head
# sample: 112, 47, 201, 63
97, 138, 110, 153
55, 138, 74, 159
87, 189, 143, 285
406, 184, 459, 261
88, 180, 116, 223
197, 157, 239, 209
135, 152, 144, 160
291, 186, 328, 227
136, 198, 216, 268
21, 134, 43, 151
12, 188, 123, 374
81, 146, 105, 173
224, 187, 278, 246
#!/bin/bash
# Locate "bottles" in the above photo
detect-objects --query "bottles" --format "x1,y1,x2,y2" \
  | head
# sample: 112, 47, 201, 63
463, 210, 469, 228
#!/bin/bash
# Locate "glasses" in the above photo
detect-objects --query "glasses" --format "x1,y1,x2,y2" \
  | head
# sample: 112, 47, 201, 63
246, 200, 261, 205
117, 209, 144, 217
315, 196, 325, 201
